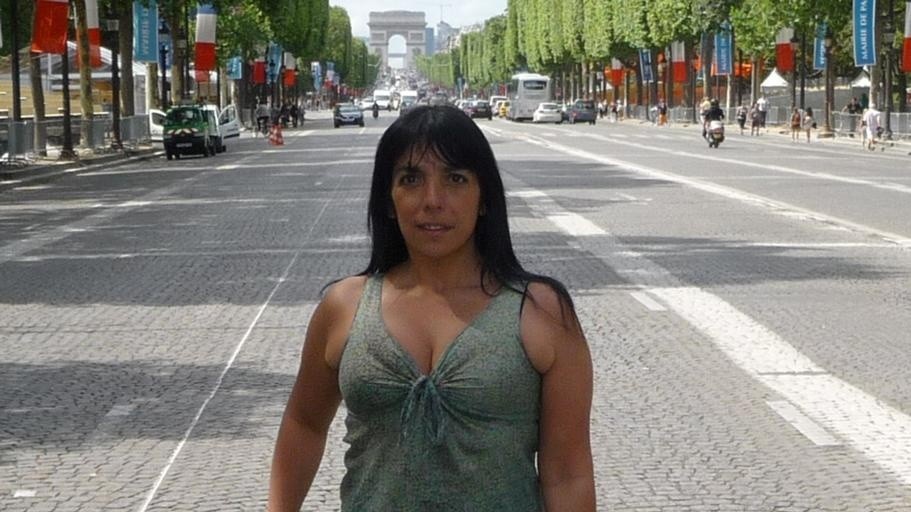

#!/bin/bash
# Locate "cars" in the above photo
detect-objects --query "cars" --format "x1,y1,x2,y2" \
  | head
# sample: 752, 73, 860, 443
332, 102, 354, 114
357, 69, 599, 126
333, 102, 366, 127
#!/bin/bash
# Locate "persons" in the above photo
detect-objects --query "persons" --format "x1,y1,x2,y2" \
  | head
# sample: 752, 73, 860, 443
264, 104, 601, 512
372, 100, 379, 112
253, 100, 307, 147
598, 93, 883, 151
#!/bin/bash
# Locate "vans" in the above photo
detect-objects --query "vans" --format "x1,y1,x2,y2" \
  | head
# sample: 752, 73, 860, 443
149, 103, 241, 160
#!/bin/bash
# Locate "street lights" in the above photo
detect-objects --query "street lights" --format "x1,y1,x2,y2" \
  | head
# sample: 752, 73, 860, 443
788, 32, 800, 114
158, 19, 172, 109
176, 26, 190, 98
596, 69, 605, 103
104, 10, 127, 151
242, 49, 356, 108
642, 47, 762, 125
816, 28, 837, 139
882, 23, 897, 141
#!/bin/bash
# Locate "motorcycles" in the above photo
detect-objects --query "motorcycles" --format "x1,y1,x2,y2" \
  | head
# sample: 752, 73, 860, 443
701, 112, 725, 149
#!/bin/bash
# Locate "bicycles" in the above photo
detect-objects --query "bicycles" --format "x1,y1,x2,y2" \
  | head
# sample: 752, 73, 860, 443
251, 117, 274, 139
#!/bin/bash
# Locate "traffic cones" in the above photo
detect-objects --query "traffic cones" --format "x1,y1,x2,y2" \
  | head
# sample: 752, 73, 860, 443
268, 122, 285, 145
